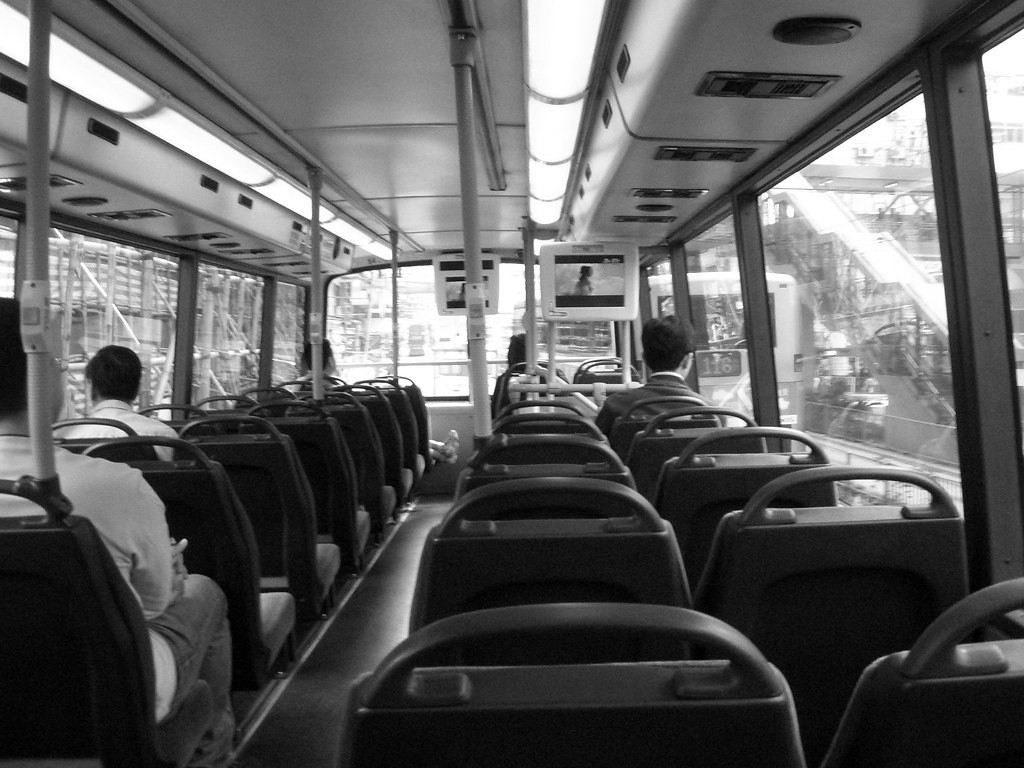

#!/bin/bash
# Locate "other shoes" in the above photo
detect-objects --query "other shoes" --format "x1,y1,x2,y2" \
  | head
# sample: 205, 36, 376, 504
447, 452, 458, 465
441, 429, 459, 458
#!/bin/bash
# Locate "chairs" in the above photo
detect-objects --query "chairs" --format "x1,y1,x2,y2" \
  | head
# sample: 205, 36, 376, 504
0, 370, 1024, 768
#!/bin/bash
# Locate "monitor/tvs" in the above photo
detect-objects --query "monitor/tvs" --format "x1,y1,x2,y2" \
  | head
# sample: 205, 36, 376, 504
540, 239, 640, 321
435, 253, 500, 316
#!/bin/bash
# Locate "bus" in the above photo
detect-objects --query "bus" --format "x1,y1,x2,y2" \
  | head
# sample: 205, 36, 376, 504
408, 324, 431, 357
647, 272, 802, 435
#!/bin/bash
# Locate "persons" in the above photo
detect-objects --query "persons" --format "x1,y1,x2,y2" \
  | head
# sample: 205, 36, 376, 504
595, 314, 726, 440
51, 342, 180, 462
490, 331, 572, 422
574, 264, 594, 295
0, 297, 235, 749
712, 317, 726, 349
288, 338, 344, 392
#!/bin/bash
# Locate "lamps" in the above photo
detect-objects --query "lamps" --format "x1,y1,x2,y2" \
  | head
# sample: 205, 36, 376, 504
0, 0, 403, 262
520, 0, 614, 227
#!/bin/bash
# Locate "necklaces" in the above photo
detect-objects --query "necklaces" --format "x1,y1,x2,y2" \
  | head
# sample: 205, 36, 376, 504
0, 433, 30, 437
